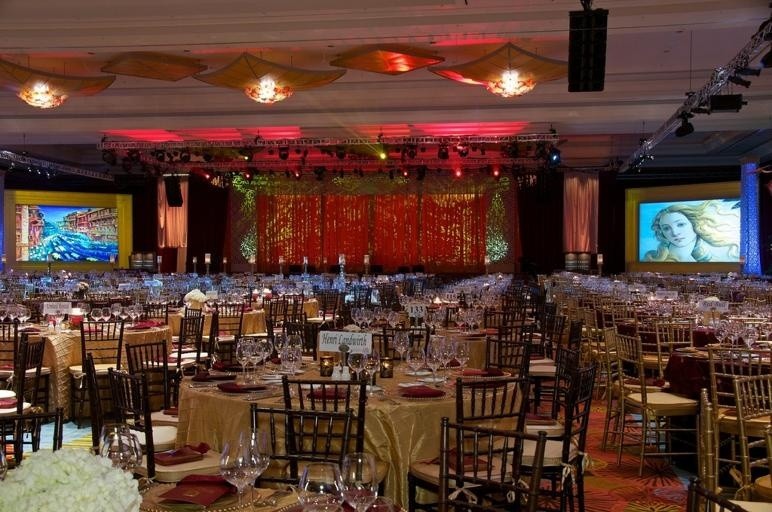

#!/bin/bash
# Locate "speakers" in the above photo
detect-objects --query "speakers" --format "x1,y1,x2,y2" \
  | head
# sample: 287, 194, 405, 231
164, 177, 181, 206
567, 9, 608, 92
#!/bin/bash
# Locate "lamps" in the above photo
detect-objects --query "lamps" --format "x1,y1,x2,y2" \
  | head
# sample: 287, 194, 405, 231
97, 133, 564, 182
1, 47, 113, 113
613, 4, 772, 176
189, 51, 345, 106
426, 43, 567, 100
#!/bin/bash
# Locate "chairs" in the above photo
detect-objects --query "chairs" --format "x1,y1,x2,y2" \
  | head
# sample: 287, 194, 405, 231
1, 252, 771, 512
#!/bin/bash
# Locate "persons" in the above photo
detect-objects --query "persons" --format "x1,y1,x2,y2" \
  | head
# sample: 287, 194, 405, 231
644, 200, 738, 262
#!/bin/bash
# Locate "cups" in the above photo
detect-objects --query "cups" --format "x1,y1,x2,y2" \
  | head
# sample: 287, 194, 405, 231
341, 452, 379, 512
0, 446, 9, 480
354, 496, 396, 512
296, 461, 344, 511
100, 423, 143, 474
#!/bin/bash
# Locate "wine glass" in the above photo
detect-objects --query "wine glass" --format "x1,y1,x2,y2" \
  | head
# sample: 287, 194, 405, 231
220, 428, 270, 512
95, 275, 347, 312
0, 302, 144, 336
351, 279, 511, 336
556, 269, 772, 360
233, 329, 472, 402
2, 273, 91, 299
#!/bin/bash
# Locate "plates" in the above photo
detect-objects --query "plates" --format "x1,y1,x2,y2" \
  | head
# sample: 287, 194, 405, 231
303, 502, 344, 512
0, 389, 17, 399
463, 371, 511, 380
